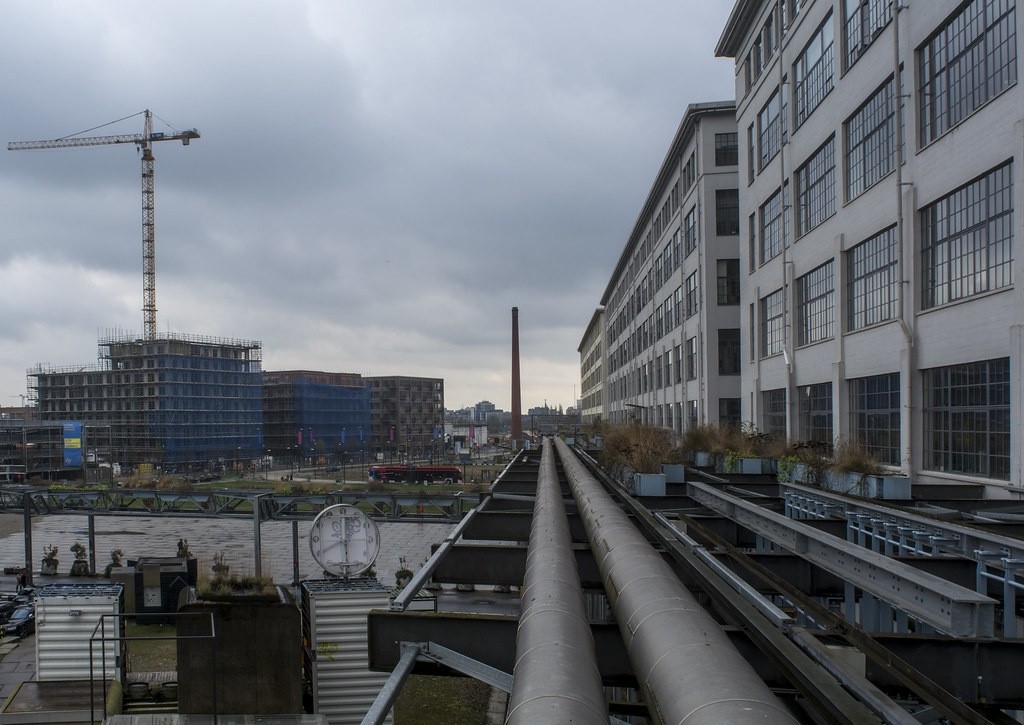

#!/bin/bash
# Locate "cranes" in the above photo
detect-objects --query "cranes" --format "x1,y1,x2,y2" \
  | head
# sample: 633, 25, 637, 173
8, 110, 201, 338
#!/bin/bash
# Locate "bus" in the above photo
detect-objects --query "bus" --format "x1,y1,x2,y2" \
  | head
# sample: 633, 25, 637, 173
367, 466, 463, 484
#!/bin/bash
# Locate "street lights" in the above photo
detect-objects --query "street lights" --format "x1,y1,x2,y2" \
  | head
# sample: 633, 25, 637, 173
293, 445, 298, 472
286, 446, 290, 471
314, 442, 317, 479
236, 447, 242, 478
261, 445, 265, 472
339, 443, 347, 484
360, 440, 366, 480
266, 449, 272, 481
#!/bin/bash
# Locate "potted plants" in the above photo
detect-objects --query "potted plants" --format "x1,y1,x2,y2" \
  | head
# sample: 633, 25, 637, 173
608, 411, 912, 505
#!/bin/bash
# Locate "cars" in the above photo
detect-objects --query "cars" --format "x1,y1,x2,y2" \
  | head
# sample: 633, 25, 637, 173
326, 467, 341, 473
181, 476, 199, 483
0, 600, 36, 639
199, 474, 222, 482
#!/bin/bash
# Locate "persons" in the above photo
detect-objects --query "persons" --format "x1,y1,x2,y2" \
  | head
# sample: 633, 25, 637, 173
16, 570, 26, 592
177, 537, 189, 553
